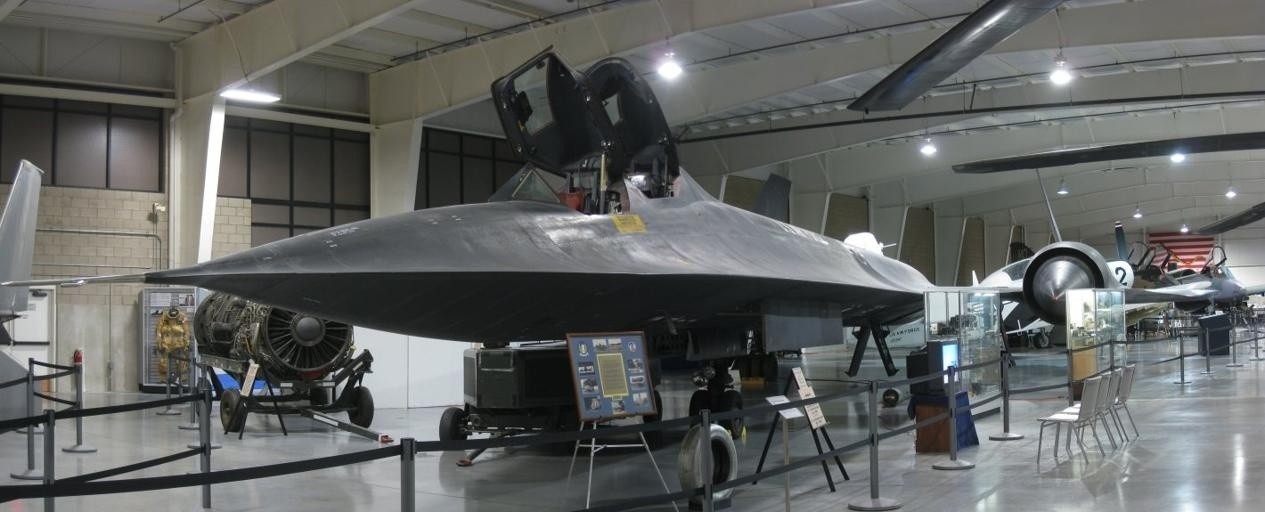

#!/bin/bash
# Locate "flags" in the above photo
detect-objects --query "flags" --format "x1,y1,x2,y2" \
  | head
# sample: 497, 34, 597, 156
1144, 232, 1213, 277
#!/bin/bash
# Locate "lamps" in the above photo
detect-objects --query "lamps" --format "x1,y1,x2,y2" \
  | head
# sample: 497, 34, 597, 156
658, 44, 683, 80
1057, 179, 1071, 195
920, 132, 938, 155
1050, 45, 1079, 87
1133, 207, 1145, 218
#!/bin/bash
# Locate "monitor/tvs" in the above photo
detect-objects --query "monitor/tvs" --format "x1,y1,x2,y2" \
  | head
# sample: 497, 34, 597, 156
907, 339, 962, 395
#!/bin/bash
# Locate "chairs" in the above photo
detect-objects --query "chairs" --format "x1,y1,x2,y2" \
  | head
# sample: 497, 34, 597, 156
1037, 366, 1136, 469
